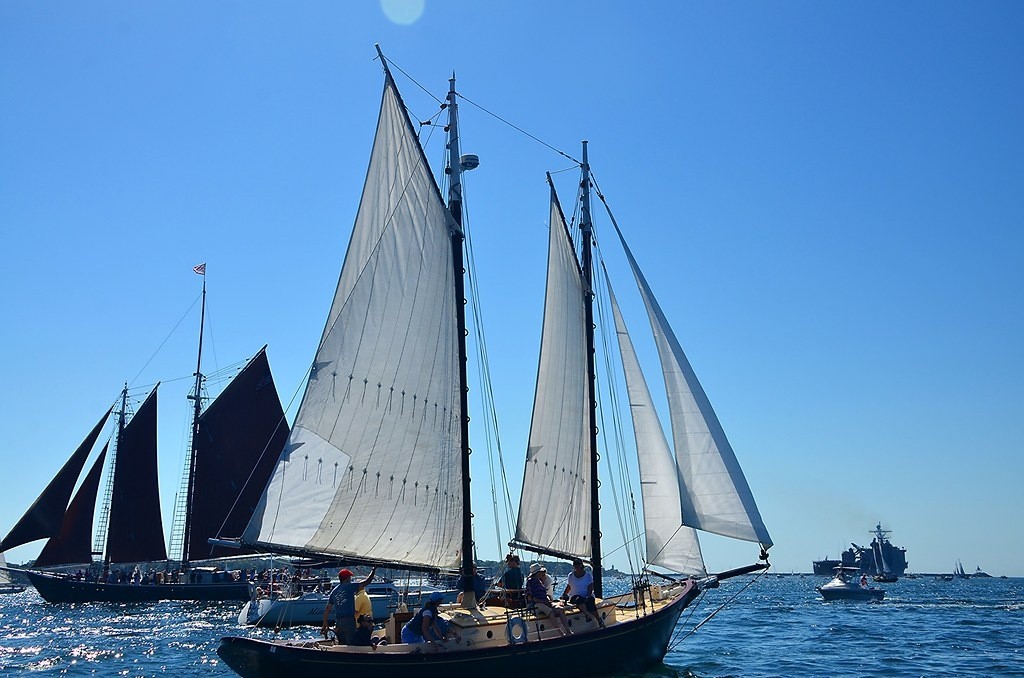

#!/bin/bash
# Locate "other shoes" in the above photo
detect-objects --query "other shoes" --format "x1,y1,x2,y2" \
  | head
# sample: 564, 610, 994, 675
557, 628, 566, 636
585, 614, 592, 622
598, 617, 604, 628
568, 629, 574, 634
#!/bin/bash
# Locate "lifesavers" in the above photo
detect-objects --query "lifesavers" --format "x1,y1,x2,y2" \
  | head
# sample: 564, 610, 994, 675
503, 616, 529, 645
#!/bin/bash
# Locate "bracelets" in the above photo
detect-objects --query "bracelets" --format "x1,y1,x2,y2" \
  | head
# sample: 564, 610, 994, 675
372, 569, 375, 570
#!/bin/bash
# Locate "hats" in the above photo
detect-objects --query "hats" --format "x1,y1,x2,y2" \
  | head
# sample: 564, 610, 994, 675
527, 563, 541, 578
506, 554, 519, 566
337, 569, 355, 578
429, 591, 446, 601
473, 561, 477, 568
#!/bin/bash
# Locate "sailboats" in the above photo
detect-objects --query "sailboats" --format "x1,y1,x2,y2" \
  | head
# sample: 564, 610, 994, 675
1, 343, 297, 599
204, 42, 776, 678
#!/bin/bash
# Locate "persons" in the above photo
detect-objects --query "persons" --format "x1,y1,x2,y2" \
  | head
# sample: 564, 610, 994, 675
456, 555, 605, 637
313, 583, 322, 593
75, 567, 180, 585
190, 570, 203, 583
237, 566, 323, 582
320, 566, 378, 646
401, 592, 448, 647
861, 574, 867, 586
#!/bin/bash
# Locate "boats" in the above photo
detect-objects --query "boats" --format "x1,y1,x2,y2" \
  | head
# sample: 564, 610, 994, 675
858, 573, 1012, 584
234, 578, 498, 626
816, 562, 887, 599
